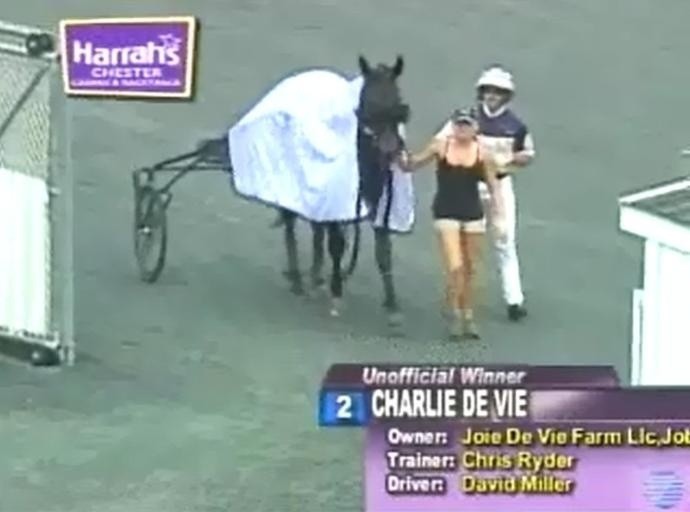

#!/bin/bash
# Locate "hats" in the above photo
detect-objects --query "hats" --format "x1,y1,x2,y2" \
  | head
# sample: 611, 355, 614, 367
450, 105, 480, 129
477, 67, 516, 96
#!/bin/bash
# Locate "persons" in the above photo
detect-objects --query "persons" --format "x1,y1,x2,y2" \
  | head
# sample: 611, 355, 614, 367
396, 107, 502, 341
434, 66, 535, 317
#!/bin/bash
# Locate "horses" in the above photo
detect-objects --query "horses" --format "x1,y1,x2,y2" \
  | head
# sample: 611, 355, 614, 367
272, 52, 408, 328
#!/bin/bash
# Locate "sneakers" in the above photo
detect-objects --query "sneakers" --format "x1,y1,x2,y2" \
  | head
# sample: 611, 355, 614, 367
507, 305, 528, 322
447, 309, 478, 340
